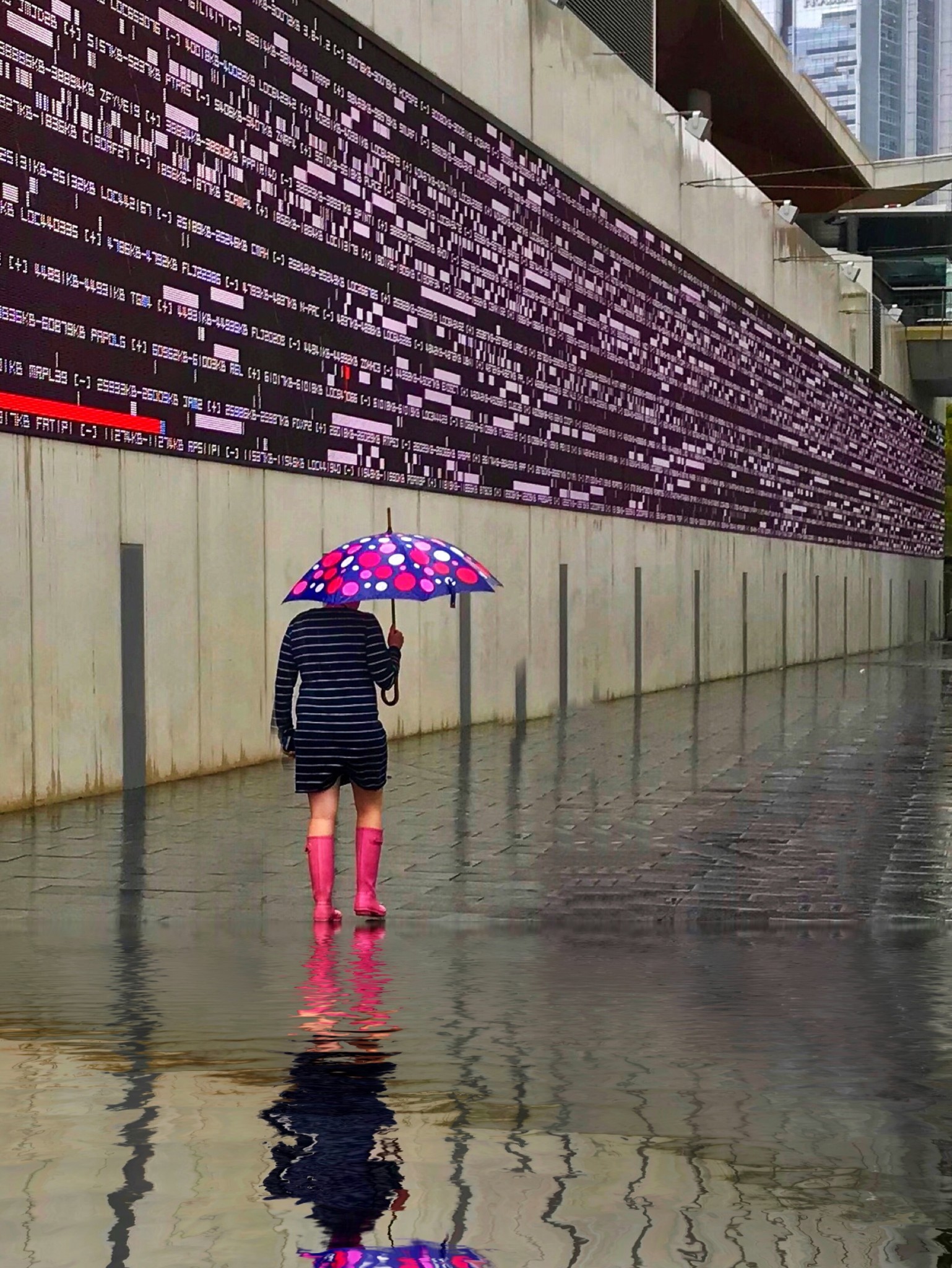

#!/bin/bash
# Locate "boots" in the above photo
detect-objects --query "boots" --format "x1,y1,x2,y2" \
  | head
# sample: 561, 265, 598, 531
304, 835, 343, 923
353, 826, 386, 918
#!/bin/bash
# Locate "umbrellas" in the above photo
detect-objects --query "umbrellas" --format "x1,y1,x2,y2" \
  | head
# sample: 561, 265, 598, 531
280, 507, 504, 707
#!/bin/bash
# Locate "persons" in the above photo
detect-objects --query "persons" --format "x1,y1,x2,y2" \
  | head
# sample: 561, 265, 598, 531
268, 601, 405, 921
